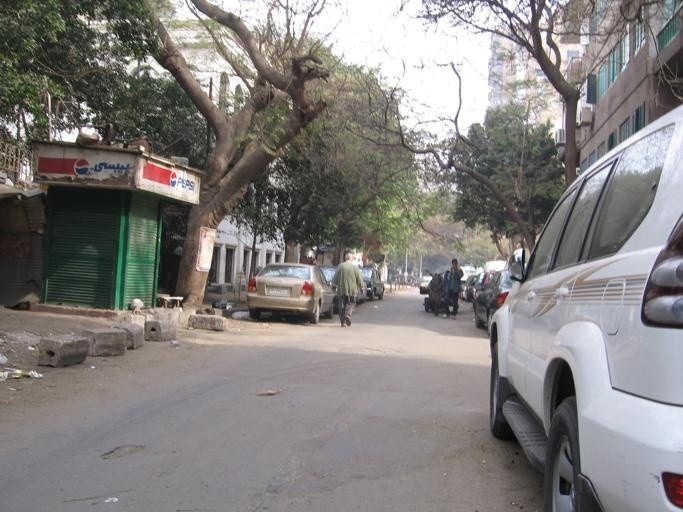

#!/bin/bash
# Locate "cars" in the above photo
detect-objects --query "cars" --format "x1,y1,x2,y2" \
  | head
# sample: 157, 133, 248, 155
246, 257, 385, 325
456, 260, 511, 337
420, 275, 432, 293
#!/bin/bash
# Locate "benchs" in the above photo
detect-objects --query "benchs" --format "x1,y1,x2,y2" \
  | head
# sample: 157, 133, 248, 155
158, 295, 183, 309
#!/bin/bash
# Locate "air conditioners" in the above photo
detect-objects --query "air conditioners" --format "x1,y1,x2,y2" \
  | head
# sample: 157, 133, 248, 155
577, 106, 592, 126
555, 127, 564, 145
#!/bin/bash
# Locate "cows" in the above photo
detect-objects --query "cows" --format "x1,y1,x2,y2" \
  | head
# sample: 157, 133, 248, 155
427, 270, 445, 316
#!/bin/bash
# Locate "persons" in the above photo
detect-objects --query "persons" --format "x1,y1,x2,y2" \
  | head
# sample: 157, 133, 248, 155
332, 252, 364, 326
443, 259, 464, 319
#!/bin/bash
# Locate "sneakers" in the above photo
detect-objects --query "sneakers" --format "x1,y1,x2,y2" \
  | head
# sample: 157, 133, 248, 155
345, 315, 351, 326
340, 324, 345, 327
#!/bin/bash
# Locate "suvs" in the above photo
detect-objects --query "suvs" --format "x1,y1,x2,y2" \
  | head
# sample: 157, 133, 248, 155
491, 106, 683, 511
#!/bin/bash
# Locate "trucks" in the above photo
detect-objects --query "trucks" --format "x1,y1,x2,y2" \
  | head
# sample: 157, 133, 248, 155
424, 272, 452, 318
